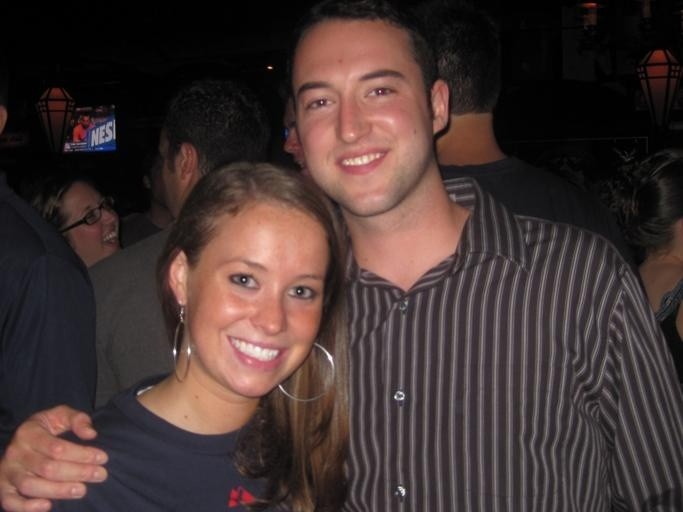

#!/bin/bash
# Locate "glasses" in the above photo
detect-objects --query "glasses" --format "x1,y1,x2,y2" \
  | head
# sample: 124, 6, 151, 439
60, 196, 115, 237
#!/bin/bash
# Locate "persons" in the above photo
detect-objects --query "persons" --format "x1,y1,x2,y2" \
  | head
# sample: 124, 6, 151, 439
1, 1, 683, 512
118, 147, 176, 250
280, 89, 311, 176
16, 161, 351, 512
27, 172, 118, 270
599, 146, 683, 387
74, 110, 96, 145
86, 75, 266, 410
425, 1, 634, 267
